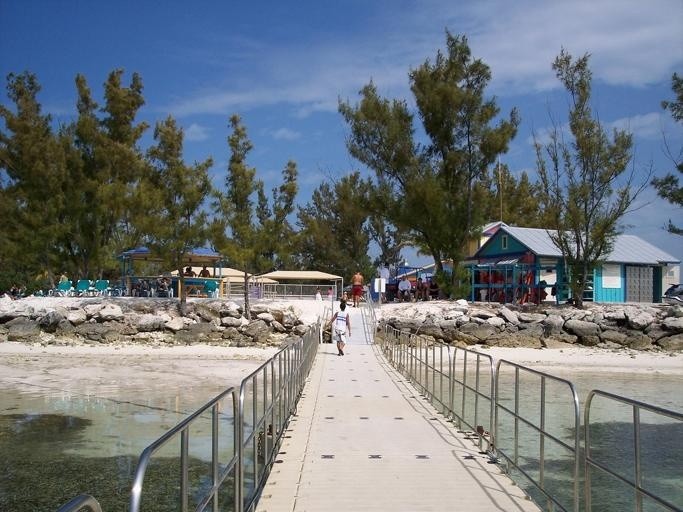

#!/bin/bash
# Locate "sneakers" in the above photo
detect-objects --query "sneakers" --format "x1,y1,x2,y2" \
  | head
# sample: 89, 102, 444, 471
337, 349, 344, 356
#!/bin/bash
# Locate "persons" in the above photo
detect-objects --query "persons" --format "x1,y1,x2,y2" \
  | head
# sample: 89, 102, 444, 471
326, 302, 352, 356
182, 264, 210, 278
108, 275, 173, 299
8, 282, 28, 298
314, 270, 440, 308
58, 272, 68, 283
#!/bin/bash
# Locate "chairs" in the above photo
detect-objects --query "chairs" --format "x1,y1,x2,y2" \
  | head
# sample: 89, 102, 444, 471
6, 277, 225, 302
